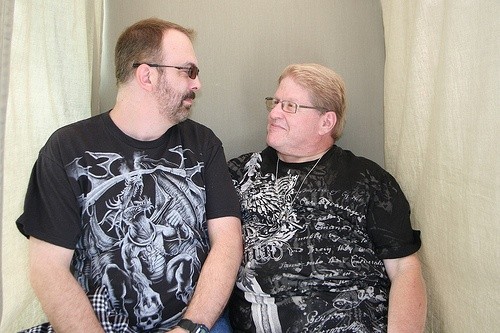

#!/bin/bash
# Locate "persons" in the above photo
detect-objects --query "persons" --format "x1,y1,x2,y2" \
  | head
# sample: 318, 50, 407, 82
227, 63, 427, 333
15, 17, 243, 333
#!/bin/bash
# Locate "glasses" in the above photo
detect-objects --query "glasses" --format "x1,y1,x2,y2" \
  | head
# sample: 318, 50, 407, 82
264, 96, 315, 113
132, 62, 199, 80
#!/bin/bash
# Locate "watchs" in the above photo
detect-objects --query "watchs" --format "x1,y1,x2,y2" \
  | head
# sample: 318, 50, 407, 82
177, 319, 210, 333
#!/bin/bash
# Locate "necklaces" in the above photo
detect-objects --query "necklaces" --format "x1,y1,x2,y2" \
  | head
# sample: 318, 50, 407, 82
275, 147, 331, 222
